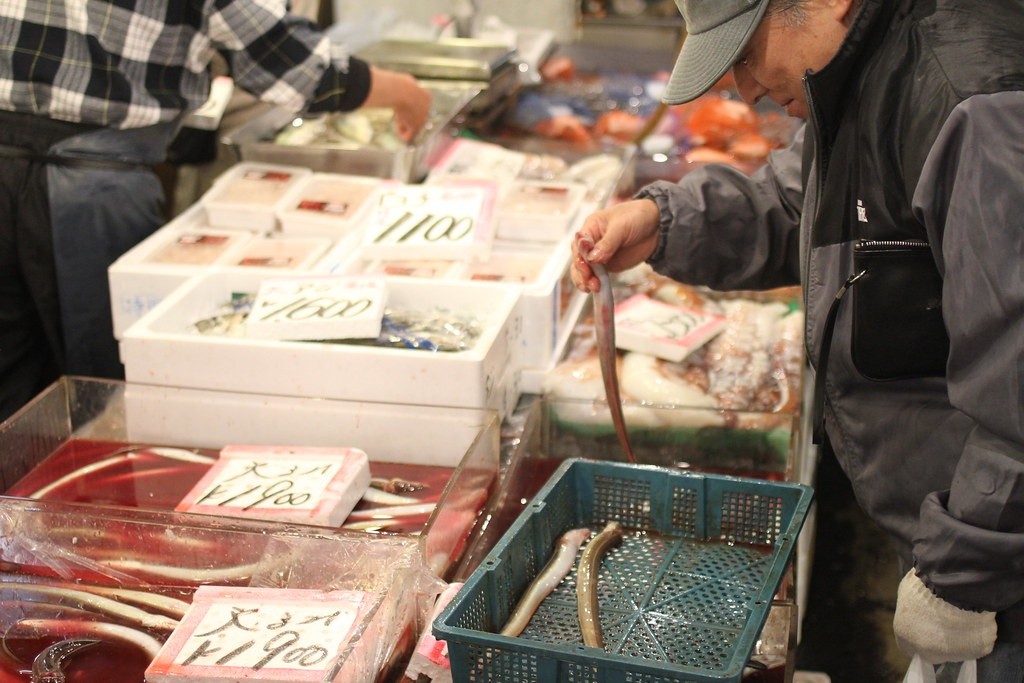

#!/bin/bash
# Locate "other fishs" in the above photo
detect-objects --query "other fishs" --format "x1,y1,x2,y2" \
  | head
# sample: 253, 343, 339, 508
578, 233, 641, 463
1, 446, 470, 672
470, 517, 632, 678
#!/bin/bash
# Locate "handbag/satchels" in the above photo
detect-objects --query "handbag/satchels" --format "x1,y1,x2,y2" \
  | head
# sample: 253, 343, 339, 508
811, 241, 947, 444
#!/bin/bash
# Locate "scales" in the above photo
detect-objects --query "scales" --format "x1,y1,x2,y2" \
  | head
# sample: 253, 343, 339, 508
347, 37, 525, 117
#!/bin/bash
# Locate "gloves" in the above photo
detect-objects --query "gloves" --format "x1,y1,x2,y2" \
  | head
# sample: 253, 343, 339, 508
892, 572, 999, 667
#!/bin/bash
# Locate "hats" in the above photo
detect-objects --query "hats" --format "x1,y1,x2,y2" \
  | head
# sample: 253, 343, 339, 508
661, 0, 772, 105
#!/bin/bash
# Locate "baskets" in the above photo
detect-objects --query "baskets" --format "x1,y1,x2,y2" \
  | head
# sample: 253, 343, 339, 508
430, 458, 815, 683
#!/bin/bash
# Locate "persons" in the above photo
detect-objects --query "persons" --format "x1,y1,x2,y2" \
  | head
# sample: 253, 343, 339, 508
570, 0, 1024, 683
0, 0, 433, 429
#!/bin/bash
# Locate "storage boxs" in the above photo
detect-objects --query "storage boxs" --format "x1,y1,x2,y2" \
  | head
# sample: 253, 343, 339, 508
429, 454, 817, 683
94, 139, 641, 436
